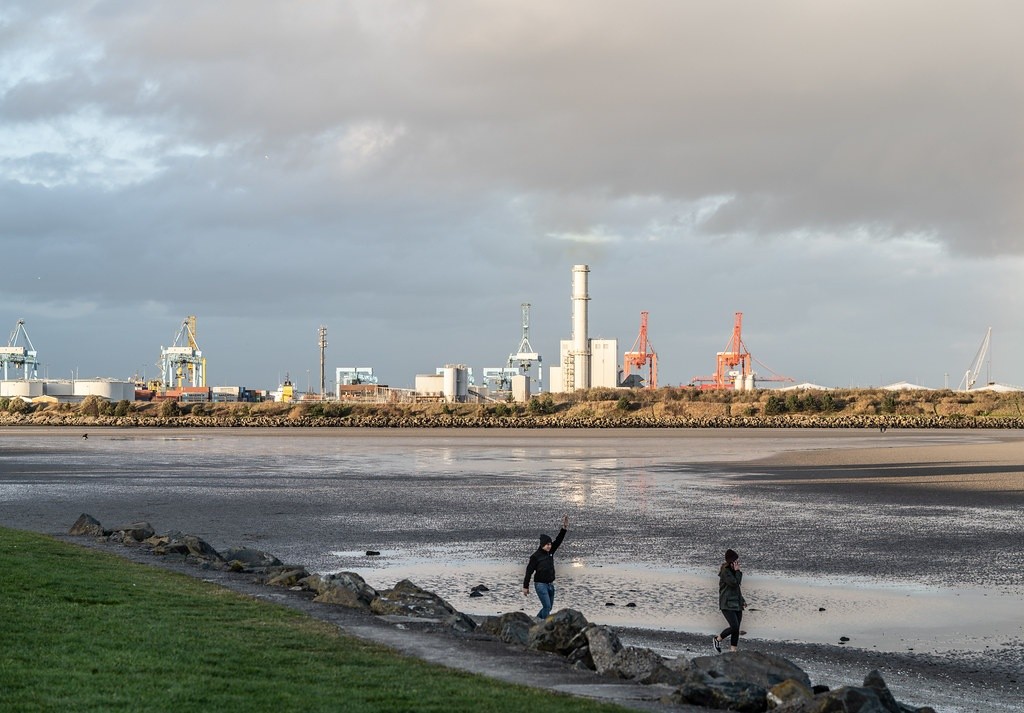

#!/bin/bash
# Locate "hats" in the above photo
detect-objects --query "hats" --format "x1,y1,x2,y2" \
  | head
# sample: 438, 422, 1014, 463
725, 549, 739, 564
538, 534, 552, 548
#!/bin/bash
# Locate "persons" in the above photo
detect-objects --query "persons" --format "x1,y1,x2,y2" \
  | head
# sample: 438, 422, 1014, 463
522, 513, 570, 619
713, 548, 749, 653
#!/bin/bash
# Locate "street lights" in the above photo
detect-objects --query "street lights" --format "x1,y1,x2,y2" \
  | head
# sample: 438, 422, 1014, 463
42, 363, 49, 379
71, 359, 79, 380
306, 369, 310, 393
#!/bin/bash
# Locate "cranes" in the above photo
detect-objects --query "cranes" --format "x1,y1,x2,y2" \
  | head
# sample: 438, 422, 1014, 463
160, 314, 206, 386
483, 304, 542, 395
959, 327, 992, 391
691, 312, 794, 391
624, 311, 658, 389
0, 319, 37, 379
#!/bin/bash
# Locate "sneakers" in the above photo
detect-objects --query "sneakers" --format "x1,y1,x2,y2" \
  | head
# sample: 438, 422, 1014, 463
713, 637, 722, 654
